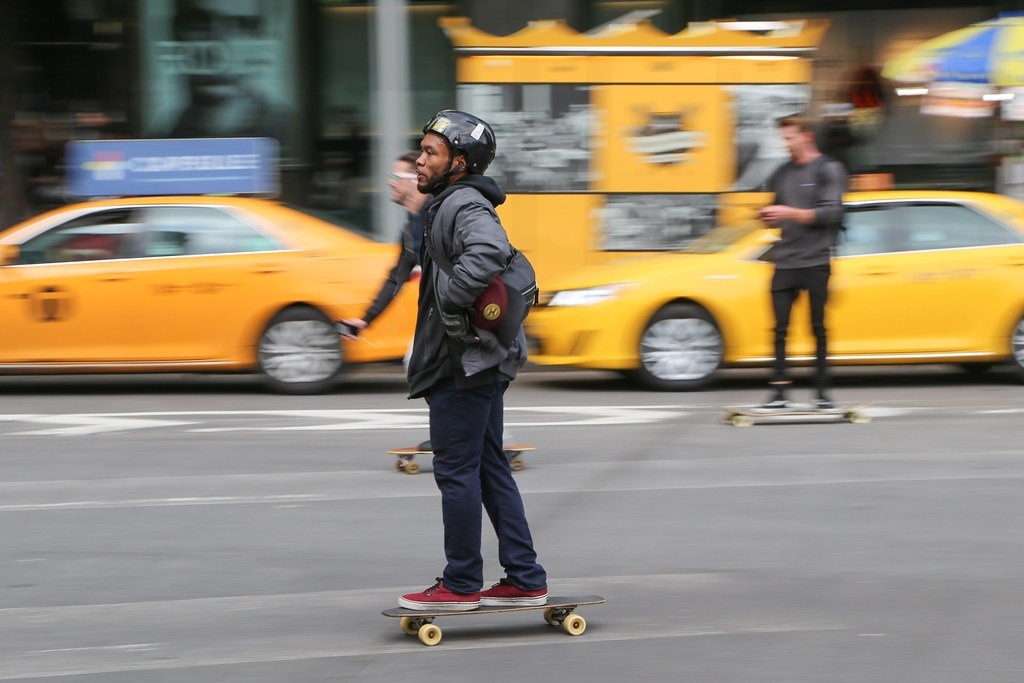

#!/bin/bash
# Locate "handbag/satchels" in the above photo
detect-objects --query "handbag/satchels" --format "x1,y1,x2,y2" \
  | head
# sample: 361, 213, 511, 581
468, 242, 540, 350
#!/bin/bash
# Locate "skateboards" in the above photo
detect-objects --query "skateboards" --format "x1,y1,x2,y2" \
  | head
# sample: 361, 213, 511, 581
380, 594, 607, 647
718, 402, 879, 427
386, 442, 538, 474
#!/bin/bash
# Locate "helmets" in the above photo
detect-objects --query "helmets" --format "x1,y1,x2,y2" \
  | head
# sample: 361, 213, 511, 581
422, 108, 497, 175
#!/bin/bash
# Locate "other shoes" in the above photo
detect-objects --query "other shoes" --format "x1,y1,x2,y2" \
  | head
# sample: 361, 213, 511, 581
418, 440, 432, 451
760, 390, 790, 407
815, 392, 836, 411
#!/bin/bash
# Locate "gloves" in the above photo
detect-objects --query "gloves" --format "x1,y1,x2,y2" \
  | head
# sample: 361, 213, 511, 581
442, 300, 480, 346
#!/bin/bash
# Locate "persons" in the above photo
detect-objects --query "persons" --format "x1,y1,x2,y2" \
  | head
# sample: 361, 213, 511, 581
335, 150, 438, 452
754, 114, 849, 403
398, 109, 549, 612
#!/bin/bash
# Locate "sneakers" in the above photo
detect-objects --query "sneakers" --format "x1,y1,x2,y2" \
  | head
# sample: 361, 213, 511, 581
480, 578, 549, 607
398, 577, 482, 611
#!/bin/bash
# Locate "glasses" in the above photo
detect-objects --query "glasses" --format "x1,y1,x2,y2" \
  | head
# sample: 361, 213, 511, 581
388, 170, 418, 181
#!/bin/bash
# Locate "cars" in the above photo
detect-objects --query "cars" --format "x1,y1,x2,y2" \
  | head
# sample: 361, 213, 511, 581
526, 190, 1024, 385
0, 136, 423, 396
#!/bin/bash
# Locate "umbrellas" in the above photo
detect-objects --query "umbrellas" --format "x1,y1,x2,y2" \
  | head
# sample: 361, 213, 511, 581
884, 11, 1024, 169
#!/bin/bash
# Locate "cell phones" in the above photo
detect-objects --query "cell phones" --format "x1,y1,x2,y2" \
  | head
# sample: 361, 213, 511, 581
334, 321, 359, 337
